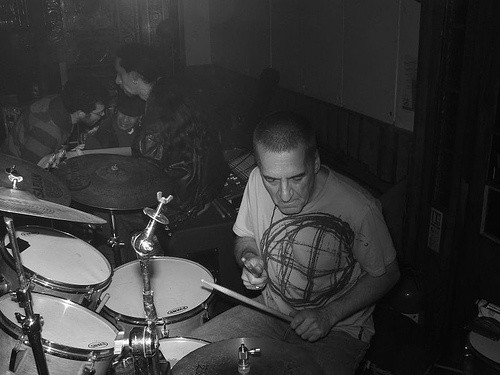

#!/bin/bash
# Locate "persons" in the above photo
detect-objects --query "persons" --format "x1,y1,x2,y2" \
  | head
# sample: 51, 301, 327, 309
190, 110, 400, 375
0, 44, 212, 265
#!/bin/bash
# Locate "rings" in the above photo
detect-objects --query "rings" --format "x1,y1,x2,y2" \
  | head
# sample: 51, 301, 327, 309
256, 286, 259, 288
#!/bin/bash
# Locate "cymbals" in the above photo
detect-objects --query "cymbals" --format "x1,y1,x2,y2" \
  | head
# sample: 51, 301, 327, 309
0, 187, 107, 224
0, 152, 72, 206
169, 337, 325, 375
52, 154, 175, 210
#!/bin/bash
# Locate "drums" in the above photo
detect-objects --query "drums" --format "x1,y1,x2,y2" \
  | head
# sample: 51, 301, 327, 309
155, 336, 213, 369
0, 225, 113, 311
99, 255, 216, 337
0, 289, 125, 375
